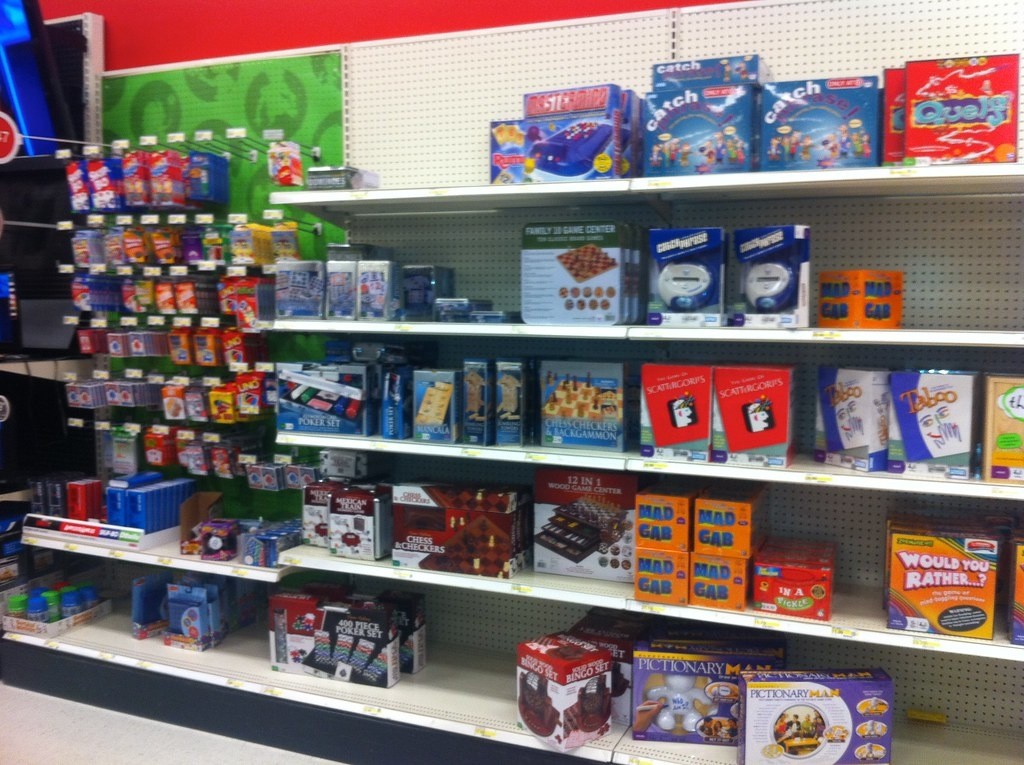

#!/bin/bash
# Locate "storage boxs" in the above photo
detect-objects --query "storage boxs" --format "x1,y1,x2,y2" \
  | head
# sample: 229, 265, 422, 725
2, 53, 1024, 765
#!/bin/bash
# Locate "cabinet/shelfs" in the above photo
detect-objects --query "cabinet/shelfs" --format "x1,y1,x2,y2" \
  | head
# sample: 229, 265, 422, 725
0, 44, 350, 749
275, 0, 1024, 765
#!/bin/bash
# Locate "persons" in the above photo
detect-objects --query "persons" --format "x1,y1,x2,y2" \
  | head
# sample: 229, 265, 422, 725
775, 709, 825, 750
630, 700, 738, 737
